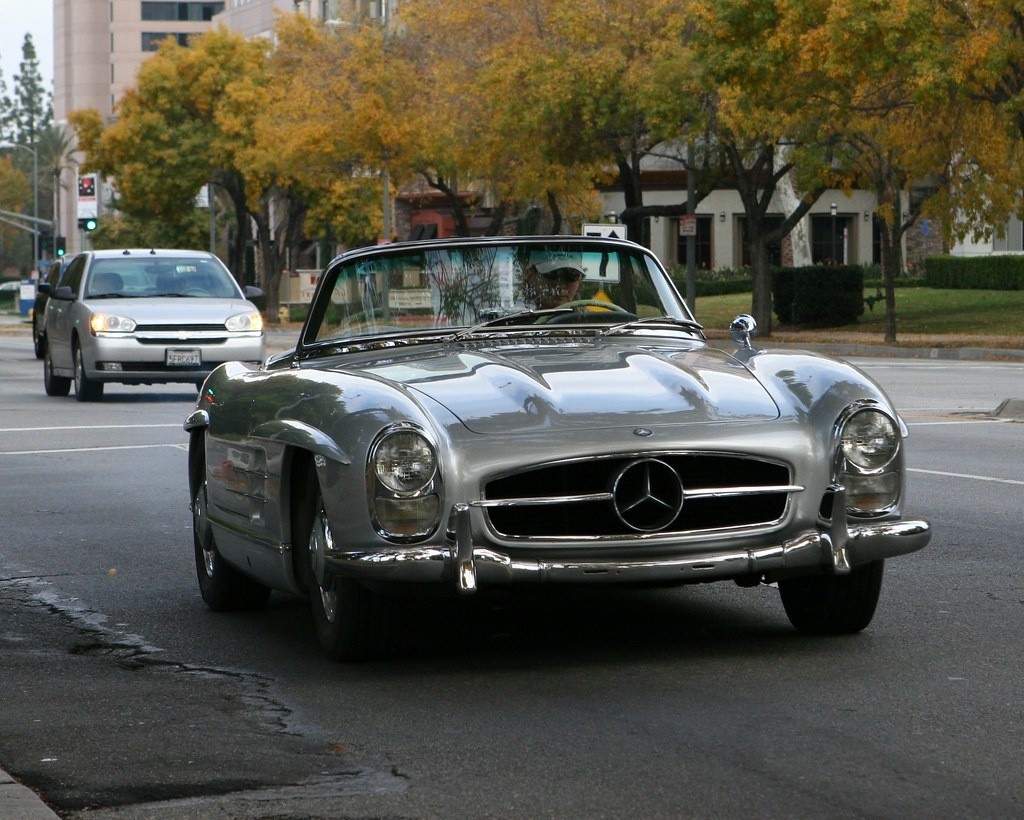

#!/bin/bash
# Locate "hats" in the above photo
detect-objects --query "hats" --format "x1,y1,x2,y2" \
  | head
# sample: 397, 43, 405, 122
528, 250, 587, 276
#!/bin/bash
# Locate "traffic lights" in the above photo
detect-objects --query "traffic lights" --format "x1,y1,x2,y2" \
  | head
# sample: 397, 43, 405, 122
56, 237, 65, 257
84, 219, 97, 231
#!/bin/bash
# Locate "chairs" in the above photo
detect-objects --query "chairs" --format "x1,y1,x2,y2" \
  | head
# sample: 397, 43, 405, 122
92, 272, 124, 293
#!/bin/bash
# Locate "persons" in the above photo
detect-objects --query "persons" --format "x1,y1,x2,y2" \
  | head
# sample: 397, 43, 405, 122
525, 247, 583, 310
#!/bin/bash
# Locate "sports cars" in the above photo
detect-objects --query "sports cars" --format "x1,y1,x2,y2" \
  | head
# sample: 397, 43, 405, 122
183, 235, 933, 661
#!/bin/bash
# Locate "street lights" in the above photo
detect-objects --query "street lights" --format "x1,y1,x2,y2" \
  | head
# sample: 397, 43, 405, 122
0, 141, 60, 260
324, 18, 392, 323
830, 203, 839, 263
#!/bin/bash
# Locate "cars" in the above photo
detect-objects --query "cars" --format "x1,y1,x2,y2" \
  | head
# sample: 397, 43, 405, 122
32, 247, 267, 401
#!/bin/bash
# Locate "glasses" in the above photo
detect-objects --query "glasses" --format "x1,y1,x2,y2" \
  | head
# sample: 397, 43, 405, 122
531, 266, 581, 283
